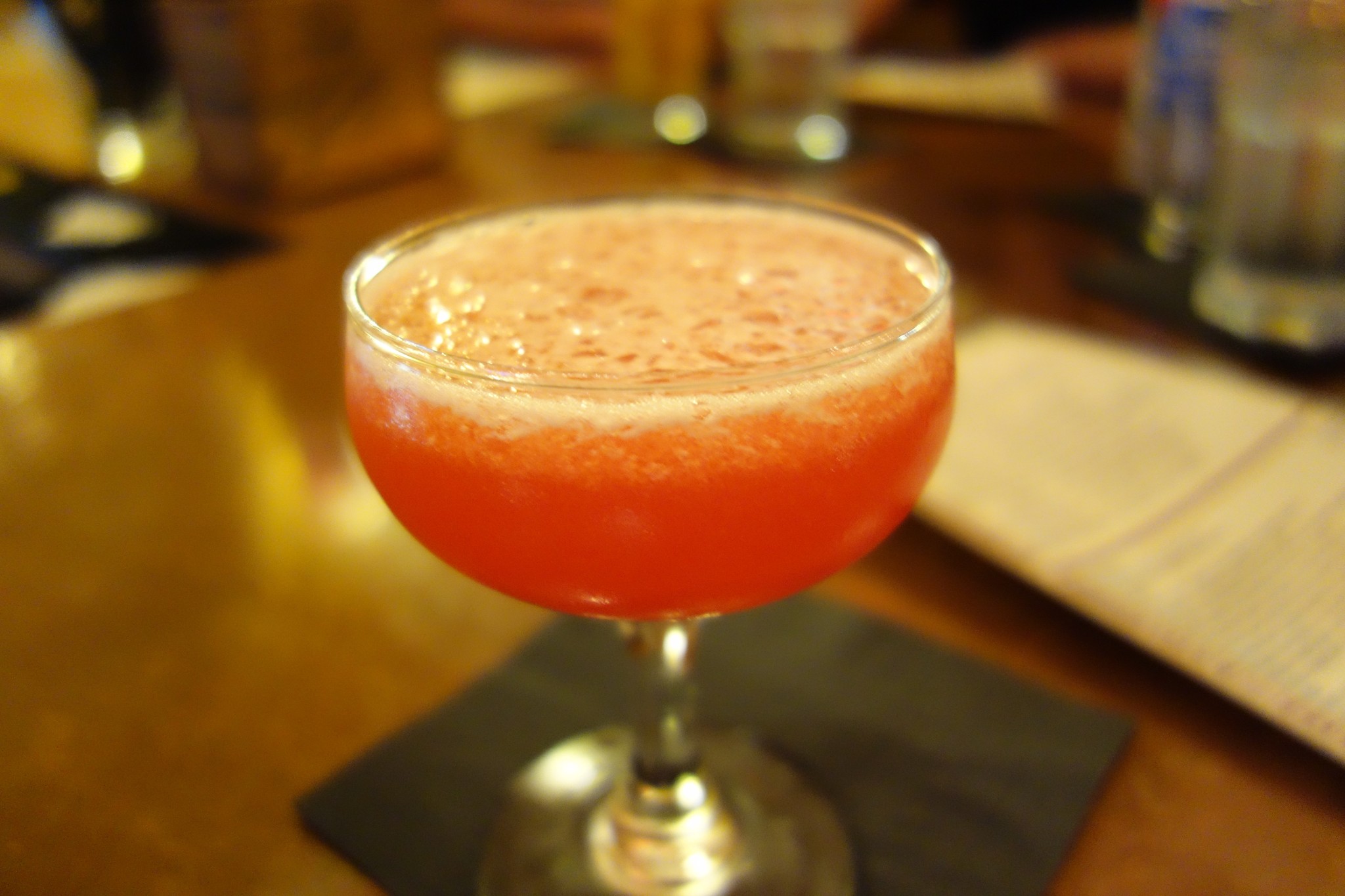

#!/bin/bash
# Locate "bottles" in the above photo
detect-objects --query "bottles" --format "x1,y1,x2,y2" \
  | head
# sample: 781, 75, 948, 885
607, 1, 852, 164
1142, 0, 1345, 349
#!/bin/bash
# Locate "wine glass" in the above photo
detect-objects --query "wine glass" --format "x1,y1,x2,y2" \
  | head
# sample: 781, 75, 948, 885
339, 184, 957, 896
